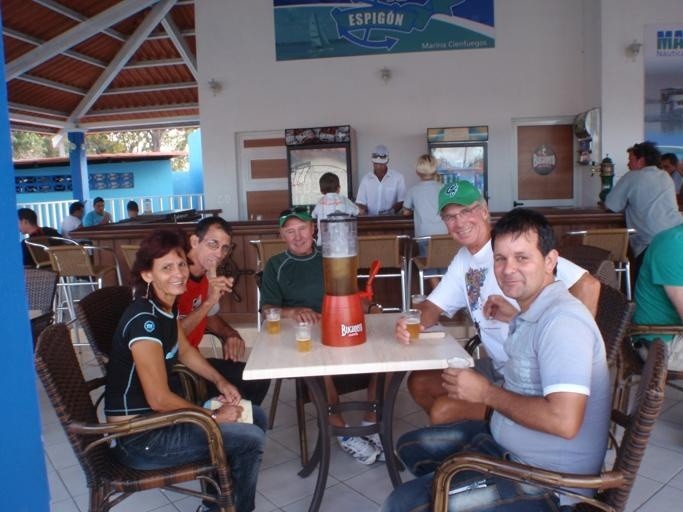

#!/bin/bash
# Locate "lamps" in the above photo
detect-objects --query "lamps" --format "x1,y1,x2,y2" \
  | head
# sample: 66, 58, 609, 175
629, 39, 643, 61
378, 65, 391, 86
207, 78, 222, 98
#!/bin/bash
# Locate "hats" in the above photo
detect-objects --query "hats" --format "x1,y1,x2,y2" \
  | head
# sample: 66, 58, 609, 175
276, 205, 312, 229
435, 180, 483, 214
370, 145, 390, 164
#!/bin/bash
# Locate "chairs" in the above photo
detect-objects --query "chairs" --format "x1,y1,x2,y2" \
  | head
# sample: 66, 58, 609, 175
18, 229, 682, 512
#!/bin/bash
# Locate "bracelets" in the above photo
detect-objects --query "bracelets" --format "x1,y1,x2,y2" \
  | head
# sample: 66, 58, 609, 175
210, 406, 219, 421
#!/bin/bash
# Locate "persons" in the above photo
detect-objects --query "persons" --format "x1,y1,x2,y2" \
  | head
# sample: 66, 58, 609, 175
258, 205, 396, 465
125, 200, 137, 218
352, 144, 404, 213
82, 196, 113, 229
395, 179, 603, 426
654, 151, 682, 213
310, 171, 367, 249
172, 216, 271, 406
627, 222, 683, 374
100, 231, 269, 511
380, 207, 612, 511
402, 154, 446, 292
61, 204, 91, 246
601, 142, 682, 303
15, 208, 59, 266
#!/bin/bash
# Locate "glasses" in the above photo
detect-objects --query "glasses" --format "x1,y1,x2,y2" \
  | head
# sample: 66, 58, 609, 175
371, 152, 387, 159
443, 205, 479, 226
279, 206, 307, 219
199, 237, 231, 253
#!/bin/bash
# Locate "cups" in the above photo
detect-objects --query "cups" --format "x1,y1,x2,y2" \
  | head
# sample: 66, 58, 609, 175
402, 308, 422, 340
264, 308, 282, 335
295, 322, 312, 350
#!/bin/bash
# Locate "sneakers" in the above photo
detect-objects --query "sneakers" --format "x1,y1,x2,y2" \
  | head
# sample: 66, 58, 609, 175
359, 419, 398, 461
335, 423, 382, 466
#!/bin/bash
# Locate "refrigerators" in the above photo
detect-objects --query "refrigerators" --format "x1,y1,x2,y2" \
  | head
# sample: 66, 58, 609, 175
282, 125, 357, 213
428, 125, 487, 204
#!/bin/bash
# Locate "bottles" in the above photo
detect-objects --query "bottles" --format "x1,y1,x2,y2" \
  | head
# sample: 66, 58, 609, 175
142, 199, 153, 215
317, 211, 365, 295
